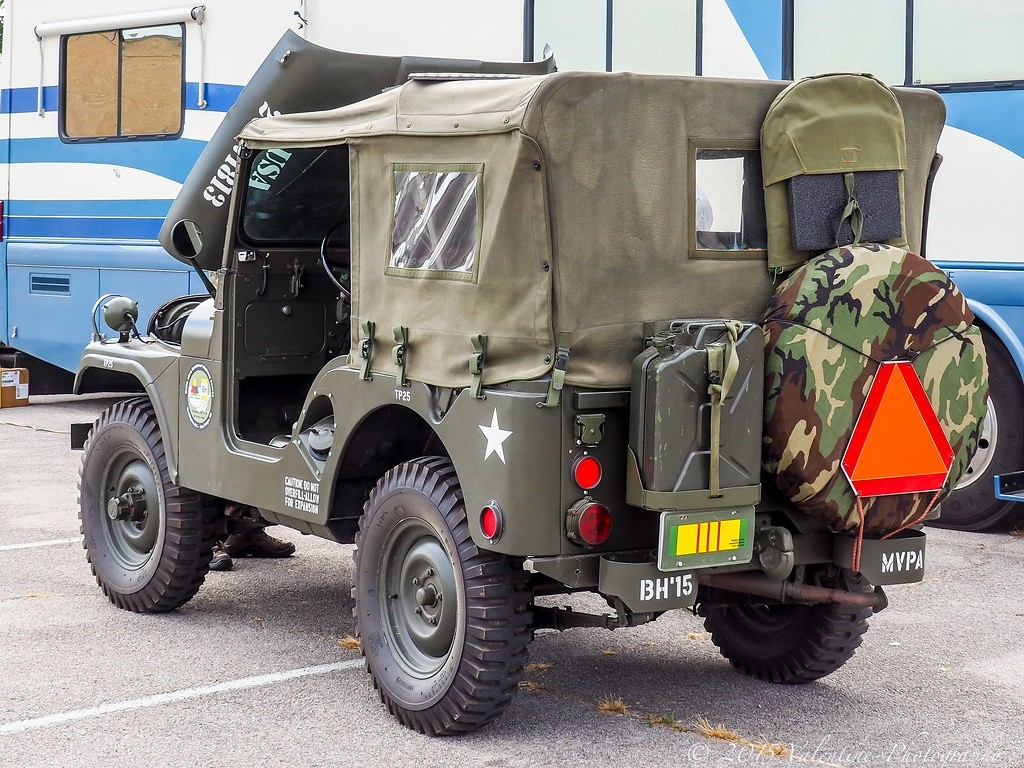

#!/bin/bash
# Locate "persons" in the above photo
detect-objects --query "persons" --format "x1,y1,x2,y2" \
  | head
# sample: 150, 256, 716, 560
208, 271, 296, 571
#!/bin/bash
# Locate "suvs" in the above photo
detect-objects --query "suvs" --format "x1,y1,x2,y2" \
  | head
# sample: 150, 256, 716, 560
70, 31, 993, 739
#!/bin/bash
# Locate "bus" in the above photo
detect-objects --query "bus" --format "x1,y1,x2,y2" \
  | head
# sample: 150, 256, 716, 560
0, 0, 1024, 533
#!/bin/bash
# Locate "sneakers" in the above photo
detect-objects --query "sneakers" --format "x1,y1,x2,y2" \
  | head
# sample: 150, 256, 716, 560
209, 526, 295, 571
